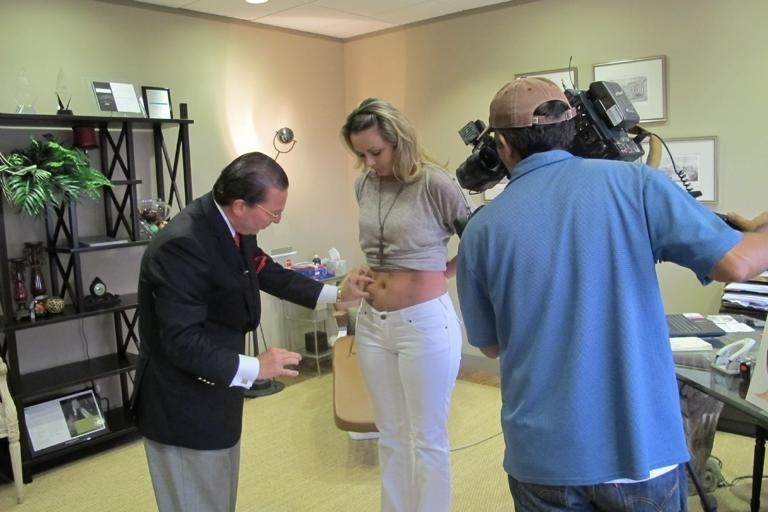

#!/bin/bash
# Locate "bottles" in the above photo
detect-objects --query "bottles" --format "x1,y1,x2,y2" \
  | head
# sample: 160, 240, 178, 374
138, 199, 169, 238
312, 254, 321, 265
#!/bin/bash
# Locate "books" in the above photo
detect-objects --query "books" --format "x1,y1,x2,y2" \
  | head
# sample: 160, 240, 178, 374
667, 336, 714, 352
719, 270, 768, 321
75, 235, 128, 247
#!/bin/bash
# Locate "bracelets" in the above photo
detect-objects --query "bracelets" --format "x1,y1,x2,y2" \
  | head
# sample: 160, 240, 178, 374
336, 284, 345, 305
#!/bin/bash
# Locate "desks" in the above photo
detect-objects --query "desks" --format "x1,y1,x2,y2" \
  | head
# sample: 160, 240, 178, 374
660, 312, 766, 512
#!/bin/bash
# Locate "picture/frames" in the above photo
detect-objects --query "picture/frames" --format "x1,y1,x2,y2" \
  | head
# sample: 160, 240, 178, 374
511, 66, 578, 95
589, 53, 669, 124
633, 134, 720, 208
21, 388, 110, 459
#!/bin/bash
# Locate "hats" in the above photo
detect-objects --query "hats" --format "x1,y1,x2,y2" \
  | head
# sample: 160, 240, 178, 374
476, 76, 578, 140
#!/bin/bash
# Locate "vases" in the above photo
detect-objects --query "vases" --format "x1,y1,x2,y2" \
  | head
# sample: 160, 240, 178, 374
23, 237, 47, 300
8, 252, 28, 307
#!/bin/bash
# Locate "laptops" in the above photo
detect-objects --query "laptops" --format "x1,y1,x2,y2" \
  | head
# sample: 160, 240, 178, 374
666, 314, 726, 338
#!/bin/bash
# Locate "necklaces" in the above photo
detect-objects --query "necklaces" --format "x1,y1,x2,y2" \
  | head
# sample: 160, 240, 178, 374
378, 176, 406, 268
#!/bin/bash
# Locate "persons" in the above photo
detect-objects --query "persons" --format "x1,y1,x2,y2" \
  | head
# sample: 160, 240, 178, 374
69, 398, 86, 422
128, 149, 375, 512
339, 96, 476, 511
457, 75, 768, 512
84, 398, 97, 415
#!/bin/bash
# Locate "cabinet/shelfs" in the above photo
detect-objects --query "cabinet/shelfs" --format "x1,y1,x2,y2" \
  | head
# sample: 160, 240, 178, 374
0, 100, 194, 485
279, 274, 354, 378
